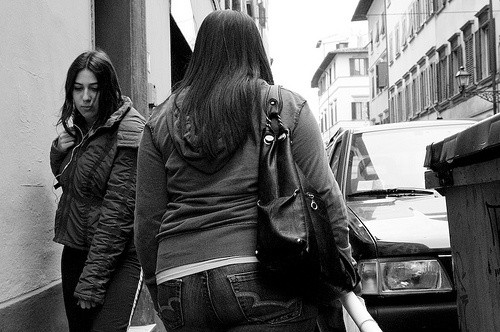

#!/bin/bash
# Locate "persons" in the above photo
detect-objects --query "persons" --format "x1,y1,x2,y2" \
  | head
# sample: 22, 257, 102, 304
134, 10, 360, 332
50, 50, 147, 332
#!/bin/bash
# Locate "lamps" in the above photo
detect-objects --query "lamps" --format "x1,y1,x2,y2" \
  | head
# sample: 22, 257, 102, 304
455, 66, 500, 104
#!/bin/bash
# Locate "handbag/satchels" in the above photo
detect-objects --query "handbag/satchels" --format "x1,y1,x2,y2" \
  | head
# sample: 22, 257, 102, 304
339, 291, 367, 331
255, 84, 362, 302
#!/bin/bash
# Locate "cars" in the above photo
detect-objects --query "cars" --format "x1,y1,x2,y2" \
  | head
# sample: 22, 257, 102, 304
312, 118, 479, 309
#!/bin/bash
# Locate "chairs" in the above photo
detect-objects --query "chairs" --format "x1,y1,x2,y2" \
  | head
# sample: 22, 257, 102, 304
352, 156, 386, 189
